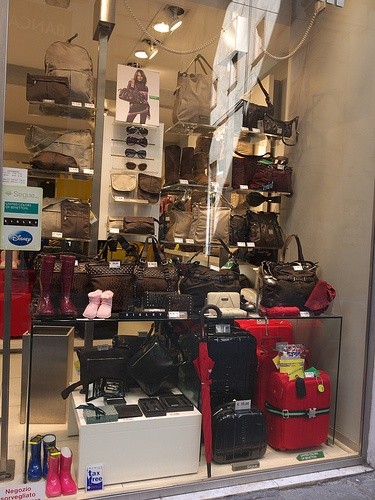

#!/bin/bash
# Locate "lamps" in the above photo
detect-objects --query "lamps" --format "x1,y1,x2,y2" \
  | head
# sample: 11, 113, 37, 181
135, 40, 160, 59
153, 6, 184, 33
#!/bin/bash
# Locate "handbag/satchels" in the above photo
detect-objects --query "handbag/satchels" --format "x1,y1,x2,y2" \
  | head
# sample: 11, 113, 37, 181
24, 124, 92, 171
119, 86, 134, 101
263, 112, 299, 146
0, 270, 35, 337
243, 77, 275, 129
172, 54, 214, 131
31, 193, 336, 319
110, 175, 136, 200
231, 149, 294, 198
25, 73, 69, 104
61, 321, 204, 402
43, 41, 93, 103
137, 173, 162, 205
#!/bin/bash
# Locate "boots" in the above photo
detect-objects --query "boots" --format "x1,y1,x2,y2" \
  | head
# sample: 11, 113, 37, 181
162, 134, 230, 189
0, 250, 20, 270
97, 290, 114, 318
60, 255, 78, 316
36, 255, 55, 316
28, 434, 77, 497
82, 289, 102, 318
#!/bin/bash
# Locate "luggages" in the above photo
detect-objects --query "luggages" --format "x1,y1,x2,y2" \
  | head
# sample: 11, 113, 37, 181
204, 319, 330, 464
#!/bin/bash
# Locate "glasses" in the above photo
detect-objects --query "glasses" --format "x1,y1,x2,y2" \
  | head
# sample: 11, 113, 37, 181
126, 136, 147, 147
125, 148, 146, 159
126, 162, 147, 170
126, 126, 148, 136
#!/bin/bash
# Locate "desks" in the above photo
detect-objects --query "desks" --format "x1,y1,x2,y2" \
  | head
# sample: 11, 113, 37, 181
67, 381, 202, 489
24, 309, 343, 480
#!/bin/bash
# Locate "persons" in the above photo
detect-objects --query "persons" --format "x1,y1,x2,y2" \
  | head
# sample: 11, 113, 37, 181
124, 70, 150, 124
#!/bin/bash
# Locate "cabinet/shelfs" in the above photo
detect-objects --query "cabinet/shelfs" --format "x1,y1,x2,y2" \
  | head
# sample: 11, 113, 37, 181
98, 115, 164, 243
160, 122, 294, 268
28, 101, 97, 243
